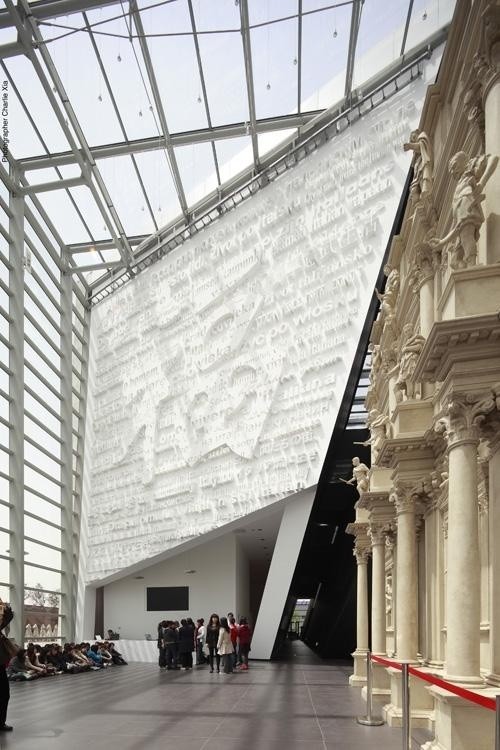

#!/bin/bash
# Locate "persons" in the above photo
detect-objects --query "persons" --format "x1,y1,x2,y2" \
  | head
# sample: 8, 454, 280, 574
347, 457, 370, 494
430, 151, 499, 265
382, 323, 425, 403
0, 599, 14, 732
5, 640, 129, 682
402, 130, 433, 204
105, 629, 116, 640
157, 611, 253, 675
369, 263, 399, 384
365, 409, 391, 451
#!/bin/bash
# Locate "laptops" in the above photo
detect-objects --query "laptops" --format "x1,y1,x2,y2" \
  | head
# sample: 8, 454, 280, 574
95, 634, 101, 639
